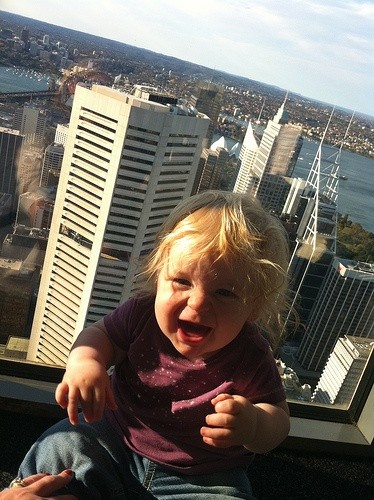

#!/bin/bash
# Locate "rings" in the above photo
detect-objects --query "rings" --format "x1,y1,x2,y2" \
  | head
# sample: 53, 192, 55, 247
12, 478, 26, 487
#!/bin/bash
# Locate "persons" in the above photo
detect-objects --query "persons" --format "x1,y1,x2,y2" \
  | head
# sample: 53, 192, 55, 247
18, 191, 292, 500
1, 468, 78, 500
0, 56, 113, 284
